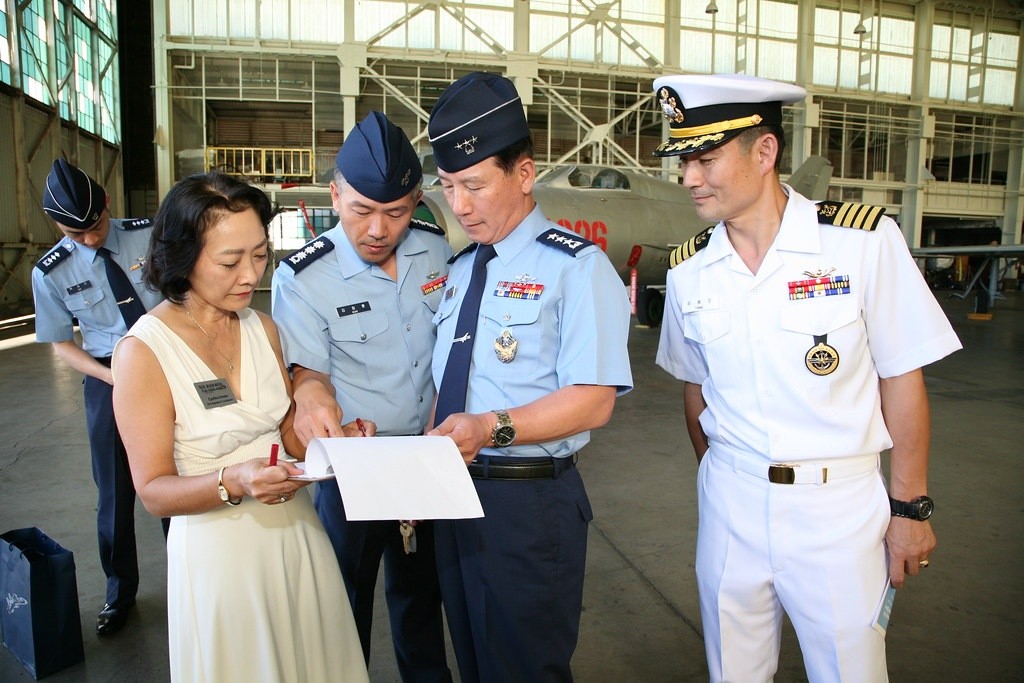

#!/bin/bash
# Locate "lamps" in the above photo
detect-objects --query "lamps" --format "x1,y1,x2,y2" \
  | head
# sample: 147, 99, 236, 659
854, 20, 866, 35
705, 0, 718, 14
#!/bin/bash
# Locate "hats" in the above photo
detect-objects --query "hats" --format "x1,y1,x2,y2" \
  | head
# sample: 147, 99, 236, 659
42, 158, 105, 230
651, 73, 806, 156
426, 71, 530, 173
336, 109, 421, 204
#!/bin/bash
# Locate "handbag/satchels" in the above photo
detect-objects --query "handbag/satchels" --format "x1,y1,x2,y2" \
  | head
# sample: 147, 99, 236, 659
0, 526, 86, 680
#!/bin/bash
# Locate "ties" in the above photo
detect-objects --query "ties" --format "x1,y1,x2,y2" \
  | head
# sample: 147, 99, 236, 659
432, 245, 498, 430
96, 247, 147, 330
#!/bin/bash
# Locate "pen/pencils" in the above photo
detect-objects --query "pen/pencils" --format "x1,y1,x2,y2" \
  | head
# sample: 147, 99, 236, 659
356, 418, 366, 437
270, 444, 279, 466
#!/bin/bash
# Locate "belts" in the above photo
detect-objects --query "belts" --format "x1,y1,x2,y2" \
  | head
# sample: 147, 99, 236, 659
466, 455, 574, 480
708, 438, 879, 485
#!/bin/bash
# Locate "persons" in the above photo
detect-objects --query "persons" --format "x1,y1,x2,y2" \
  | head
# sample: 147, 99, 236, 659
271, 111, 454, 683
31, 159, 166, 636
425, 72, 634, 683
654, 75, 964, 683
110, 172, 377, 683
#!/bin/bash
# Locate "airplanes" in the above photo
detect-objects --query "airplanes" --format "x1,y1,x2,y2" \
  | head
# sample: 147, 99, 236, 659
412, 155, 834, 327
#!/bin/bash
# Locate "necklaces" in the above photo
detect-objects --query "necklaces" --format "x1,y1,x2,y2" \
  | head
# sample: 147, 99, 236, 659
184, 307, 233, 369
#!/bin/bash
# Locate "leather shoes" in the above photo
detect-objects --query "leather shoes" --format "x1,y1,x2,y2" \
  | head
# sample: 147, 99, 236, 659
94, 602, 135, 636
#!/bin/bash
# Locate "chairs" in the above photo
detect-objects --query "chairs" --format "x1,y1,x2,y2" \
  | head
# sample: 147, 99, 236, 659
274, 171, 288, 184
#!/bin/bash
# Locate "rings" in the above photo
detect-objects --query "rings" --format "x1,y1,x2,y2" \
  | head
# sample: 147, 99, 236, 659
278, 494, 288, 503
918, 558, 929, 568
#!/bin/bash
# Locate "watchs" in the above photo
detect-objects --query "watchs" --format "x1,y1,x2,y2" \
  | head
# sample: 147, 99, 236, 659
218, 467, 243, 506
888, 494, 934, 522
490, 410, 516, 449
